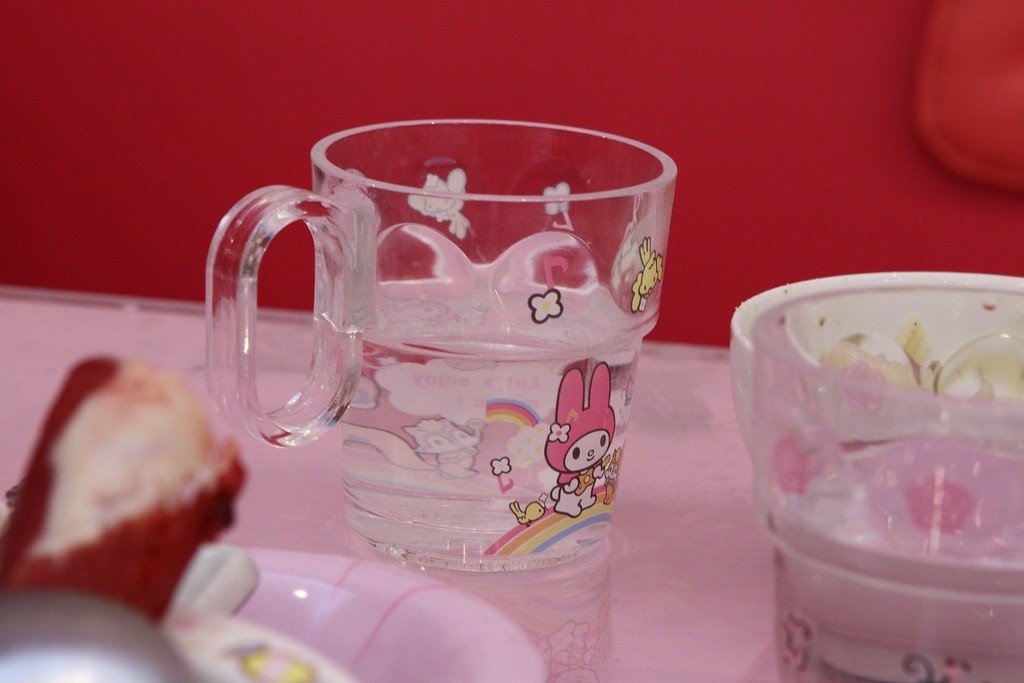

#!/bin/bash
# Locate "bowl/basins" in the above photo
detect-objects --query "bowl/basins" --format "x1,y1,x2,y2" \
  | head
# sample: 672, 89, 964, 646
730, 272, 1024, 582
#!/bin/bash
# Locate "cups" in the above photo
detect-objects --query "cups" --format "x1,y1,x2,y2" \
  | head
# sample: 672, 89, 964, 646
205, 117, 677, 574
746, 282, 1024, 683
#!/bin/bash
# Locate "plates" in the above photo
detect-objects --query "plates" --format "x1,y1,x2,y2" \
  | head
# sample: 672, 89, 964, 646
247, 547, 550, 683
